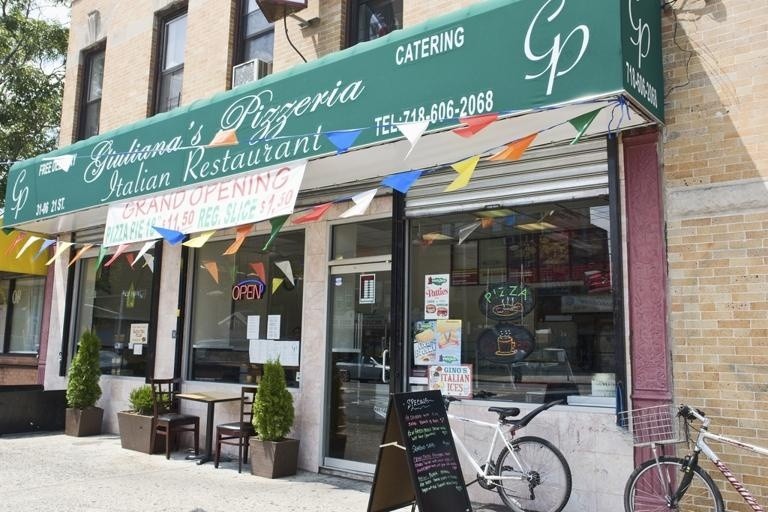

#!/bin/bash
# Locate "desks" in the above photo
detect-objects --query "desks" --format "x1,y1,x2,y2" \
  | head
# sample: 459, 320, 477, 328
173, 391, 247, 466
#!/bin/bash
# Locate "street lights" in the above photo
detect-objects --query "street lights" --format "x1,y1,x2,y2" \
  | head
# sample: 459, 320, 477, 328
83, 288, 145, 358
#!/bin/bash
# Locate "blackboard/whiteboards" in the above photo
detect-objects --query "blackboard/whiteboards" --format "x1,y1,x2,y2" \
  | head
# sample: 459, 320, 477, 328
479, 283, 535, 321
367, 390, 472, 512
477, 324, 536, 364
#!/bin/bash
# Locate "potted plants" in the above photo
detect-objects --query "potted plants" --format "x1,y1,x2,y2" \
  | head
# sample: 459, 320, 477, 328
118, 385, 174, 455
62, 329, 106, 437
248, 357, 299, 478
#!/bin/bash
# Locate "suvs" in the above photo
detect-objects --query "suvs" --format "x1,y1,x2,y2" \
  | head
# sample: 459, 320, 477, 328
331, 345, 390, 381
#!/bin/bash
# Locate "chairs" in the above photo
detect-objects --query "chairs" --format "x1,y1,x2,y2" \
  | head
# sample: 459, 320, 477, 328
145, 375, 200, 460
215, 387, 257, 474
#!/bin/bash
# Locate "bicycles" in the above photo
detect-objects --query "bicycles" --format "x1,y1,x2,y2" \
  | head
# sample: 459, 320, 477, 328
411, 390, 572, 512
617, 398, 768, 512
